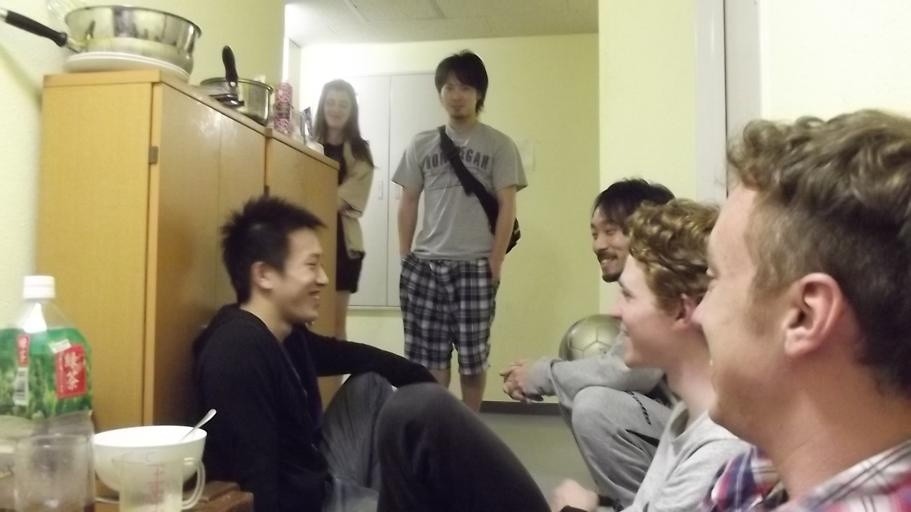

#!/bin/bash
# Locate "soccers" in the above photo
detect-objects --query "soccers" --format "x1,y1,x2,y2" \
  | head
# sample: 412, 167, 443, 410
559, 315, 622, 361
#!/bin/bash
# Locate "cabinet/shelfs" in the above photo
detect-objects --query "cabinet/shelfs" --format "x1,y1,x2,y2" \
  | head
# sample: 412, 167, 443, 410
37, 66, 271, 498
267, 130, 348, 422
88, 472, 255, 512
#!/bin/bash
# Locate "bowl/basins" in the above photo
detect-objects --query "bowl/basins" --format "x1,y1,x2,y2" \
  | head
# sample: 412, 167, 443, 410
62, 6, 201, 50
85, 421, 210, 493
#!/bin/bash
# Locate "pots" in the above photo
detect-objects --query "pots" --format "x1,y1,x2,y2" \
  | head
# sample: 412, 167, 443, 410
0, 7, 195, 73
200, 43, 273, 123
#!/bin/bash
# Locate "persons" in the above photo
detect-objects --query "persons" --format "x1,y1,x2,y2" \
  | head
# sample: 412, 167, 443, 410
308, 81, 374, 339
191, 195, 439, 512
691, 109, 911, 512
373, 195, 753, 512
390, 49, 528, 416
500, 178, 677, 508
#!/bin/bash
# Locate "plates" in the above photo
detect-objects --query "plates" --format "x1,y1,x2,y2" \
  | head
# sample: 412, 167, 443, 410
60, 51, 191, 84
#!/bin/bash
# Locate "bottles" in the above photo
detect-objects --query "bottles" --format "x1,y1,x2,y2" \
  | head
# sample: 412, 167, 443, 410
2, 277, 97, 510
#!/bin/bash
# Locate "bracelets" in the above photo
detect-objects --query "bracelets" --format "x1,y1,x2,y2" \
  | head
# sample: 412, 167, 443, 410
558, 506, 587, 512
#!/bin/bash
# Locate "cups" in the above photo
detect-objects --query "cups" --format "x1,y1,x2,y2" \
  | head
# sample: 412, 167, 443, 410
1, 409, 96, 511
112, 448, 206, 511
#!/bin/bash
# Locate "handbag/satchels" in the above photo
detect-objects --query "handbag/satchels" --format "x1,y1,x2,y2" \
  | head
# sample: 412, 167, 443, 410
437, 123, 520, 254
